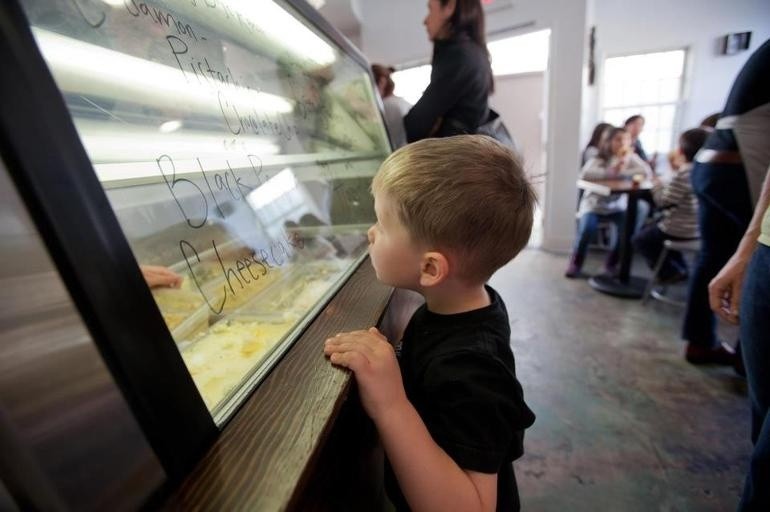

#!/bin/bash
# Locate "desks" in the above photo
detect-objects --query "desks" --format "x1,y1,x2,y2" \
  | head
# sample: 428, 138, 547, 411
576, 176, 654, 298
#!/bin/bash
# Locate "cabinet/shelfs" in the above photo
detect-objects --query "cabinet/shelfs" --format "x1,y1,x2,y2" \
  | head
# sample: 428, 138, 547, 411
1, 0, 401, 510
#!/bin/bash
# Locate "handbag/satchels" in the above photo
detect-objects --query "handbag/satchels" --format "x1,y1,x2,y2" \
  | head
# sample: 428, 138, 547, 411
470, 107, 522, 162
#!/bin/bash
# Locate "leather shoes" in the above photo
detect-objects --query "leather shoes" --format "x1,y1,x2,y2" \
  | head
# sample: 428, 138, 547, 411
686, 338, 750, 382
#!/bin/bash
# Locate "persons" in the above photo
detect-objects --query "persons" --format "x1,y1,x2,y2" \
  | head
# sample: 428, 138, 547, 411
139, 264, 183, 288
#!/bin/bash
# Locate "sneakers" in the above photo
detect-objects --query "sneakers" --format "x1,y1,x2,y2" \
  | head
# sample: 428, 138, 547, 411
565, 262, 620, 279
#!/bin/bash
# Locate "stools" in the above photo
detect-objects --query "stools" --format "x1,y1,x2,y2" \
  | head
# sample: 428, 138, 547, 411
642, 237, 702, 314
